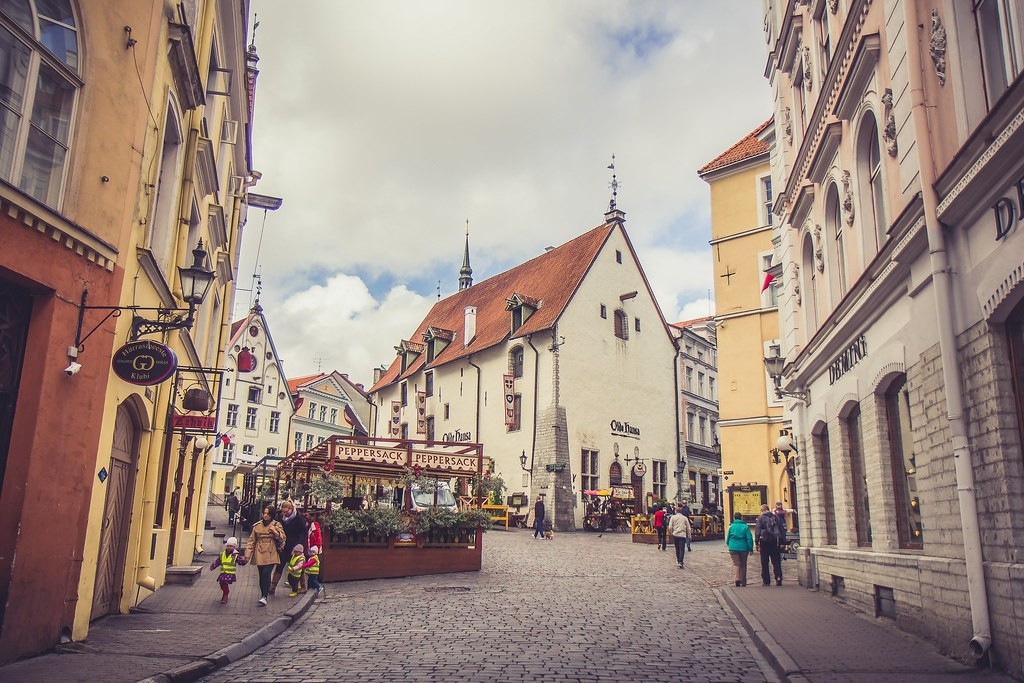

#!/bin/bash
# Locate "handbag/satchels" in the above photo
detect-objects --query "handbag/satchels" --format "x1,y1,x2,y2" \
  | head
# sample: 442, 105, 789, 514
234, 496, 240, 512
273, 521, 285, 553
662, 511, 671, 526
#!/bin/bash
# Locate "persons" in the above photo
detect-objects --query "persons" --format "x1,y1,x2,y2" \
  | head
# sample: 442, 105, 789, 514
287, 544, 306, 597
302, 545, 324, 595
244, 505, 286, 606
755, 504, 786, 586
668, 507, 691, 568
209, 537, 244, 604
284, 513, 322, 586
771, 501, 787, 528
268, 500, 309, 594
654, 500, 692, 551
225, 485, 242, 527
531, 496, 545, 539
726, 512, 753, 587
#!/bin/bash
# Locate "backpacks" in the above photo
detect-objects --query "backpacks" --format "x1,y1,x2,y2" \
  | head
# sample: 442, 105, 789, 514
760, 514, 781, 540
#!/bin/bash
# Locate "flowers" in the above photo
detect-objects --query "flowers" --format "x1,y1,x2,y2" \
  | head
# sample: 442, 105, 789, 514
251, 466, 504, 585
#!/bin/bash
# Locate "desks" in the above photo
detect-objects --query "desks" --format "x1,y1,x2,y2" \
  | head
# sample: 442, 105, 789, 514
472, 505, 509, 530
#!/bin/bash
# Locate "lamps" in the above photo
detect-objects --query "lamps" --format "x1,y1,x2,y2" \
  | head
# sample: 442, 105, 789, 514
519, 450, 532, 474
764, 342, 811, 407
771, 447, 780, 464
131, 236, 214, 341
673, 455, 687, 477
712, 434, 721, 463
191, 437, 207, 463
777, 435, 795, 480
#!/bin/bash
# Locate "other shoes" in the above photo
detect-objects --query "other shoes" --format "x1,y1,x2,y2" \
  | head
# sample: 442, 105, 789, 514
658, 544, 661, 550
288, 592, 297, 598
676, 562, 683, 567
532, 534, 535, 539
762, 583, 770, 586
318, 587, 323, 594
736, 581, 746, 587
259, 598, 268, 604
776, 582, 782, 586
221, 599, 228, 604
284, 580, 290, 586
539, 537, 544, 539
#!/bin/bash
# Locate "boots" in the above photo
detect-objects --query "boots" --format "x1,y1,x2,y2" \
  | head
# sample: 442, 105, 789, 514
268, 571, 282, 595
298, 572, 308, 594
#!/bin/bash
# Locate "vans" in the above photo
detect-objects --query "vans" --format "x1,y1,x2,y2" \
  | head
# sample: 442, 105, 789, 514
371, 480, 460, 516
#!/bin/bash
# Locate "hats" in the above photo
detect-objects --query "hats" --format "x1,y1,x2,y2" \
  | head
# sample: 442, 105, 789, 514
226, 537, 237, 547
310, 545, 318, 554
293, 544, 303, 552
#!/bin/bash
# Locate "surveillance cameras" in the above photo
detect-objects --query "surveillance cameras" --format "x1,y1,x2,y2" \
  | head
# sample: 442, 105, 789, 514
64, 363, 81, 376
